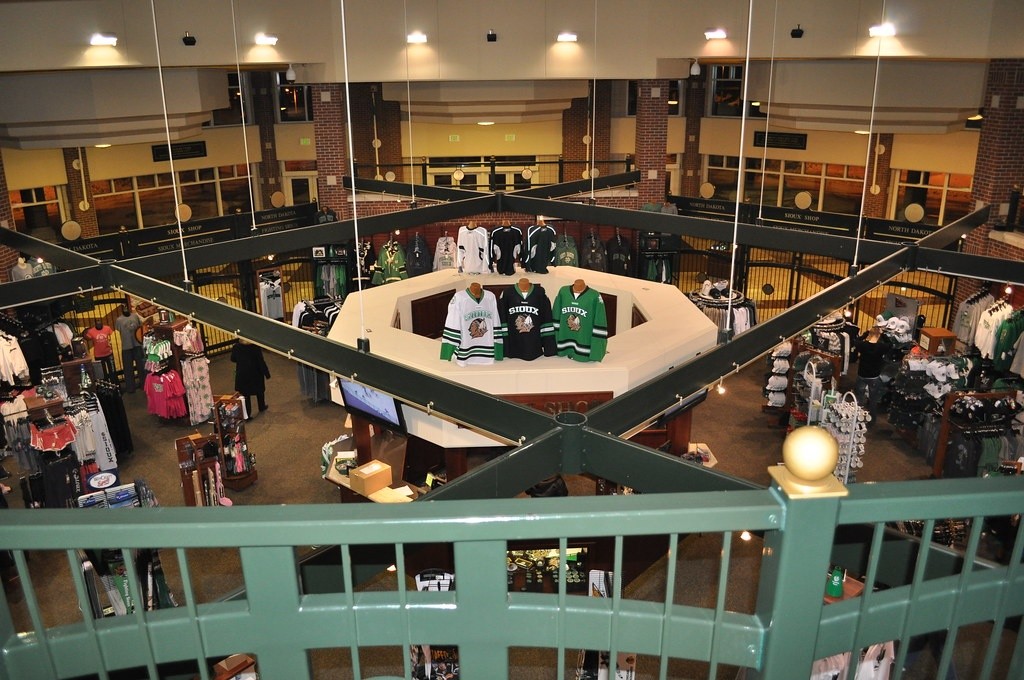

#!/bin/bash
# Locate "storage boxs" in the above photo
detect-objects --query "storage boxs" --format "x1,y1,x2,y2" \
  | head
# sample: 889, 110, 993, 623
218, 653, 246, 672
349, 460, 392, 497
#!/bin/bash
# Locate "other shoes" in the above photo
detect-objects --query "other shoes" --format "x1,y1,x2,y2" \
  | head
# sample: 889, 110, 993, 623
259, 404, 268, 412
247, 415, 253, 421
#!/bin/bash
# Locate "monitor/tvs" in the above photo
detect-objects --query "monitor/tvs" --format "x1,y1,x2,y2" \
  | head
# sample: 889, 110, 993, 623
336, 376, 408, 436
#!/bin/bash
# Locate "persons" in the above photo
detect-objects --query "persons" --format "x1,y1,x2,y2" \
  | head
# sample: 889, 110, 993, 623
552, 279, 608, 363
499, 278, 558, 360
86, 319, 121, 390
115, 307, 146, 393
231, 339, 270, 422
855, 326, 892, 427
11, 257, 33, 281
27, 257, 56, 277
456, 220, 556, 276
439, 282, 503, 366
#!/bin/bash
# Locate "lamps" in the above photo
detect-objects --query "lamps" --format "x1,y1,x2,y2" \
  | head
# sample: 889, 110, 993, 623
486, 29, 497, 43
90, 31, 118, 47
790, 23, 805, 38
556, 30, 577, 42
182, 30, 196, 47
253, 32, 279, 45
703, 27, 727, 41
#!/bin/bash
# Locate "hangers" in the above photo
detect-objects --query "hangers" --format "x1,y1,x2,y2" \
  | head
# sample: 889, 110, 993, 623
143, 323, 208, 383
965, 424, 1017, 440
0, 379, 122, 431
301, 296, 342, 315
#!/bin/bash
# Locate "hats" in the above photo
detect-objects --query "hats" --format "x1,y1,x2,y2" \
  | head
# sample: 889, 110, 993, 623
767, 391, 786, 408
874, 310, 913, 347
766, 374, 788, 391
881, 347, 983, 430
771, 342, 792, 357
772, 358, 790, 374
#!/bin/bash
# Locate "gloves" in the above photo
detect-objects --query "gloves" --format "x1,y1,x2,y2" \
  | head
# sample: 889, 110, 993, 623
266, 374, 271, 379
